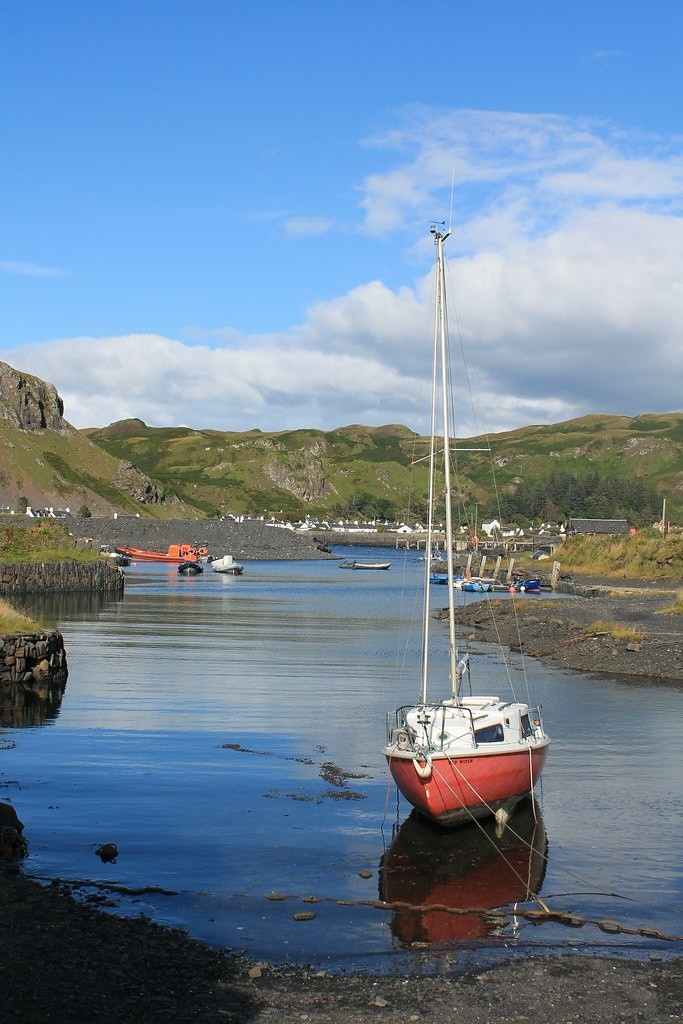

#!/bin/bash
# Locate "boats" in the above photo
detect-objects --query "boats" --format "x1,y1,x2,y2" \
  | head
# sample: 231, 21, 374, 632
97, 546, 132, 568
338, 561, 392, 570
210, 555, 244, 574
176, 559, 203, 574
429, 569, 546, 594
118, 545, 206, 564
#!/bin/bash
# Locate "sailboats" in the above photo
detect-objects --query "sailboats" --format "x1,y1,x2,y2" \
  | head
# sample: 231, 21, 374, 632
374, 795, 551, 953
383, 216, 550, 826
